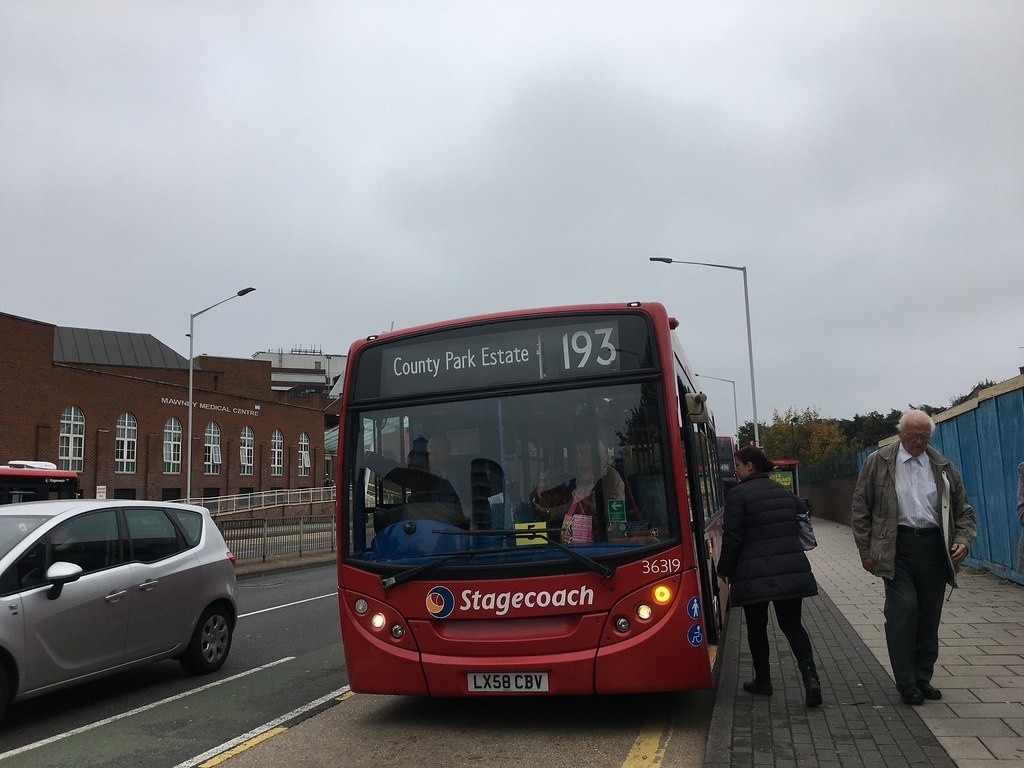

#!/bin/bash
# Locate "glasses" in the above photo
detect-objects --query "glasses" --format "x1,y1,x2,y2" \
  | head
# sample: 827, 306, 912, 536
902, 430, 931, 442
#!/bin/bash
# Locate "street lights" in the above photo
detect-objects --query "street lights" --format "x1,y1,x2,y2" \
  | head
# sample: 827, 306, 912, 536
694, 373, 740, 451
789, 415, 800, 459
649, 257, 761, 450
185, 287, 257, 505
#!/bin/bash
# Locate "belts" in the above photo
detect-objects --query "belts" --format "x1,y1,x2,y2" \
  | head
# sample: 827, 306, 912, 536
898, 525, 939, 536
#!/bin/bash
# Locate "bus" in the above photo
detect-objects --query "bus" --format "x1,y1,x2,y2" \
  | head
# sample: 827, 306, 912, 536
715, 435, 739, 501
0, 461, 82, 505
334, 302, 732, 698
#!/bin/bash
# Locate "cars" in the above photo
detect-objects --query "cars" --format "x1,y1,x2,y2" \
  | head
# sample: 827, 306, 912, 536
0, 498, 239, 731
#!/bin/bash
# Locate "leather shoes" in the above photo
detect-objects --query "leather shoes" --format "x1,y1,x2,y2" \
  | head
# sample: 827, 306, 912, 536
896, 683, 924, 704
918, 682, 941, 700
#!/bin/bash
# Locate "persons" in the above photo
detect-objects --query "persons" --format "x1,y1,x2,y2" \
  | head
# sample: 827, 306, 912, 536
717, 447, 823, 706
325, 474, 330, 487
1016, 462, 1024, 572
531, 437, 626, 546
850, 411, 978, 706
374, 433, 479, 530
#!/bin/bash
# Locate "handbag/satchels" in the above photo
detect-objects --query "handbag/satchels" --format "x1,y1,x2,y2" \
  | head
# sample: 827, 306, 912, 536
790, 489, 818, 551
560, 497, 594, 545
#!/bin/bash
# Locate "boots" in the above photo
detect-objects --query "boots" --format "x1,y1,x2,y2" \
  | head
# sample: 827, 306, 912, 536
743, 656, 772, 696
799, 659, 822, 706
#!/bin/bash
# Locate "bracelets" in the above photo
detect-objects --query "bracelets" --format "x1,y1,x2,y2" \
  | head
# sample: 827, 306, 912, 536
546, 510, 549, 516
